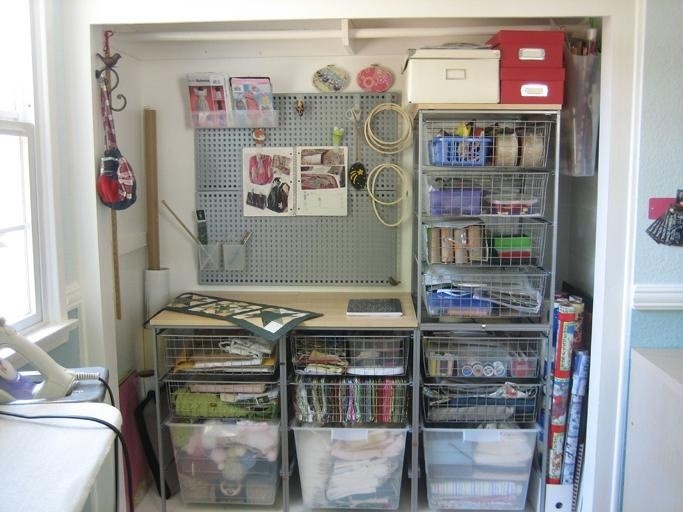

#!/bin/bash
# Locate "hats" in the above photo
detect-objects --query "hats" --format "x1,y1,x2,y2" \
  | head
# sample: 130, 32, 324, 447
349, 163, 366, 188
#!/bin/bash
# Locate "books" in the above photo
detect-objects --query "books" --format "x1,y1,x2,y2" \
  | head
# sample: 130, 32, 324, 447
348, 297, 403, 318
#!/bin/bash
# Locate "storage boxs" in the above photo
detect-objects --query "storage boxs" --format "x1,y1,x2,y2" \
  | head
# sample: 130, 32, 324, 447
428, 137, 492, 166
401, 49, 500, 110
425, 344, 540, 379
429, 191, 480, 217
292, 419, 410, 509
499, 67, 566, 105
423, 275, 492, 316
485, 29, 564, 67
419, 413, 540, 512
164, 416, 279, 506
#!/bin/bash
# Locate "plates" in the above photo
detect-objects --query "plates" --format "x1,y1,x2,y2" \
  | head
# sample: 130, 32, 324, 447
357, 66, 392, 92
313, 66, 349, 91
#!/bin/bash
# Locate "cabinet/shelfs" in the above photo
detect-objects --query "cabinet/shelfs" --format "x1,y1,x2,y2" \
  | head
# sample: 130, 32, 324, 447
417, 110, 554, 327
151, 324, 287, 512
416, 327, 553, 511
281, 328, 416, 512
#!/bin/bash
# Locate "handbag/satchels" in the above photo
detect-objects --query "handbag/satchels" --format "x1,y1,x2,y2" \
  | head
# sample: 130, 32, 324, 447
245, 188, 266, 210
266, 177, 290, 213
97, 76, 137, 210
